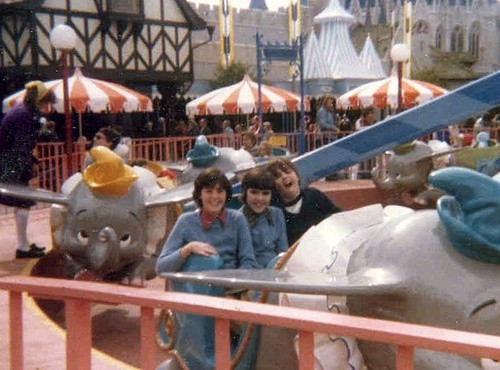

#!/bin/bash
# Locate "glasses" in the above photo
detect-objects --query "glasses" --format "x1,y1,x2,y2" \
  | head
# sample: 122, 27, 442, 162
95, 135, 104, 140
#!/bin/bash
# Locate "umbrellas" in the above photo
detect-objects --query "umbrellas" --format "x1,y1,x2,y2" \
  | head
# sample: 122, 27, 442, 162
336, 76, 448, 109
3, 68, 152, 138
185, 73, 311, 130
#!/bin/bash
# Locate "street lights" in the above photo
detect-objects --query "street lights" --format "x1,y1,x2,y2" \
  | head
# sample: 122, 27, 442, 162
49, 25, 78, 180
390, 43, 411, 113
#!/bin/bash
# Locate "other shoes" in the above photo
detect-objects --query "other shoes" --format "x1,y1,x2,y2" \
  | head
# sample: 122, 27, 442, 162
30, 243, 46, 251
16, 249, 45, 258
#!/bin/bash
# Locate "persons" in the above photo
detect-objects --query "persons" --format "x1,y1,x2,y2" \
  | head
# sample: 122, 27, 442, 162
303, 97, 376, 180
420, 107, 500, 147
153, 157, 341, 337
112, 112, 273, 160
0, 81, 119, 258
